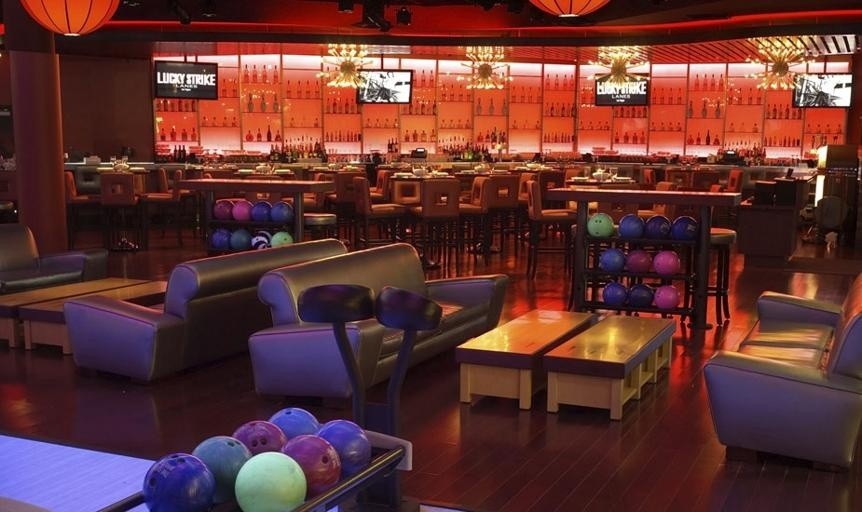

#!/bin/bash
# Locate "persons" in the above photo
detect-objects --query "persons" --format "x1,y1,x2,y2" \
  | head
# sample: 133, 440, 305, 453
372, 71, 398, 103
813, 75, 839, 106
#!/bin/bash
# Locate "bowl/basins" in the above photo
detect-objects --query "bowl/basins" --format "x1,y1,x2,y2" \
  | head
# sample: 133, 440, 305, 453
591, 172, 608, 181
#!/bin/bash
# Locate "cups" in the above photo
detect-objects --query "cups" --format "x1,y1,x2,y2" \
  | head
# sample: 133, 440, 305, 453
609, 168, 618, 182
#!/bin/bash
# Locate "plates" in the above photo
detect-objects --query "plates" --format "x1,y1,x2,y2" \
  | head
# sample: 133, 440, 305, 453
236, 168, 294, 176
615, 177, 632, 183
459, 165, 509, 175
570, 176, 589, 183
526, 163, 540, 169
392, 167, 452, 182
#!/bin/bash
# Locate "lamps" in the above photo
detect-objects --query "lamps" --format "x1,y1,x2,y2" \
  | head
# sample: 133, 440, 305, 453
744, 35, 818, 91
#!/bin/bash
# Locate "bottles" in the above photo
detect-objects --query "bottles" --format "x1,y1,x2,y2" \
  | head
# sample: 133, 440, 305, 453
386, 69, 577, 152
172, 143, 187, 163
202, 61, 322, 128
388, 84, 578, 197
269, 141, 314, 164
579, 73, 843, 168
156, 92, 197, 113
159, 126, 196, 143
325, 63, 508, 128
247, 124, 366, 155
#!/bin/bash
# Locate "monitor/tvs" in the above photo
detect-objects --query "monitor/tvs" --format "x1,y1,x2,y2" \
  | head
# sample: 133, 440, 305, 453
594, 73, 649, 106
154, 61, 218, 99
356, 69, 413, 103
793, 72, 854, 108
